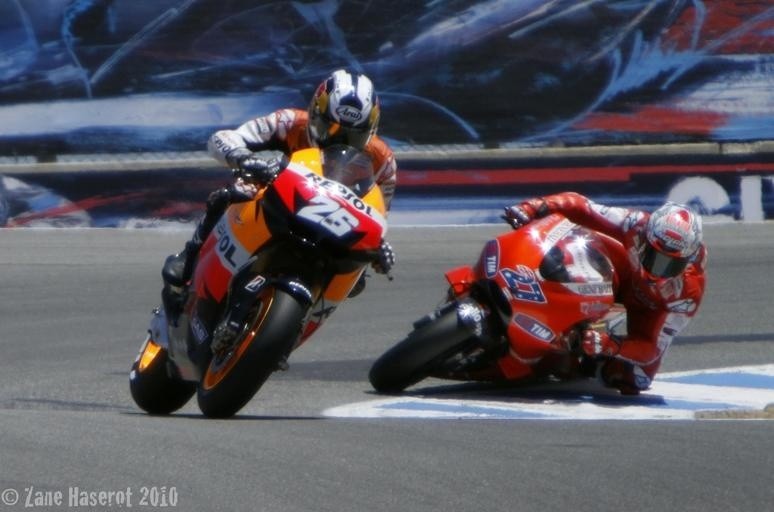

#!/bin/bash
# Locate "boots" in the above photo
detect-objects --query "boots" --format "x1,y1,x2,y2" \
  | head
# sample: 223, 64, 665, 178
163, 239, 201, 287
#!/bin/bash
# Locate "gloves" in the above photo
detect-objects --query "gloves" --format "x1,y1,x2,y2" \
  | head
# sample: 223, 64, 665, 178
370, 240, 395, 274
238, 150, 289, 180
582, 329, 620, 357
507, 196, 547, 228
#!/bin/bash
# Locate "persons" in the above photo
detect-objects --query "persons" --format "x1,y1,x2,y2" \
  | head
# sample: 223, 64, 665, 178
161, 69, 396, 357
503, 189, 706, 396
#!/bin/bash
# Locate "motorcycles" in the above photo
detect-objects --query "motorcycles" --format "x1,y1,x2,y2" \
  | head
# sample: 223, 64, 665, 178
128, 147, 394, 418
366, 209, 640, 401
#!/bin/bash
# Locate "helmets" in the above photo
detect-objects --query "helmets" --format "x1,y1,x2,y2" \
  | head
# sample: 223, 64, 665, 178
644, 200, 703, 278
308, 69, 381, 153
